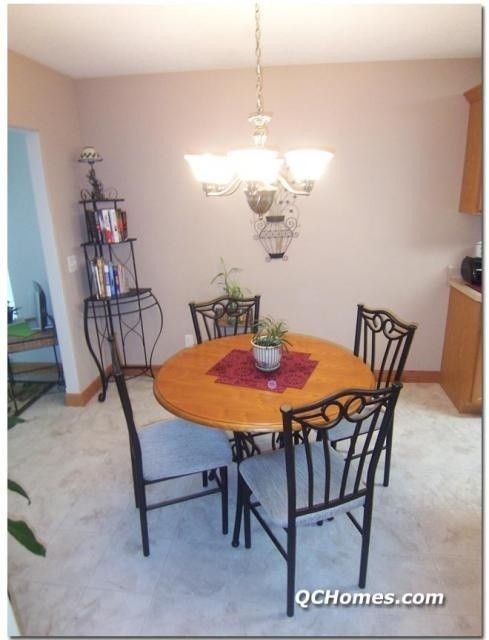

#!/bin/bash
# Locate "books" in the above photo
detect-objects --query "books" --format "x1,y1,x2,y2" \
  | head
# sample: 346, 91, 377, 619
86, 207, 127, 300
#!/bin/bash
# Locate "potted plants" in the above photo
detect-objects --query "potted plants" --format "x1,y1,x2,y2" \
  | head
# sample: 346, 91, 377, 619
208, 254, 249, 325
249, 316, 290, 375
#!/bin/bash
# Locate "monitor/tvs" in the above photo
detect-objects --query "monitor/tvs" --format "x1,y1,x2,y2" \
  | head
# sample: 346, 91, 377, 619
31, 281, 53, 332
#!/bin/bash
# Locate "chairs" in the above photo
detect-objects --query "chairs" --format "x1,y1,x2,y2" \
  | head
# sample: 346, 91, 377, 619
102, 335, 234, 556
229, 381, 407, 619
302, 301, 421, 491
181, 295, 266, 479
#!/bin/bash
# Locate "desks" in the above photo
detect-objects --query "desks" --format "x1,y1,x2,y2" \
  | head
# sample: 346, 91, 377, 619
8, 317, 59, 352
153, 330, 383, 550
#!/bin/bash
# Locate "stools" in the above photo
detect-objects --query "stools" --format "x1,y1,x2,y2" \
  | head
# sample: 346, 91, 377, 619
212, 307, 254, 339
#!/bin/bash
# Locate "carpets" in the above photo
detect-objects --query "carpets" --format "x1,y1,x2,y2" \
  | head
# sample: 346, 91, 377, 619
9, 380, 62, 423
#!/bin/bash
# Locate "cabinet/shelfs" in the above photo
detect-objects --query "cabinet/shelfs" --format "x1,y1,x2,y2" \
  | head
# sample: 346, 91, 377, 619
79, 207, 166, 404
441, 276, 482, 416
453, 83, 483, 214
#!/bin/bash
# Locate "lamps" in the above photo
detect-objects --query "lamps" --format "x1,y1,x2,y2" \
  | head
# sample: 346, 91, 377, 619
181, 7, 338, 214
75, 143, 110, 197
